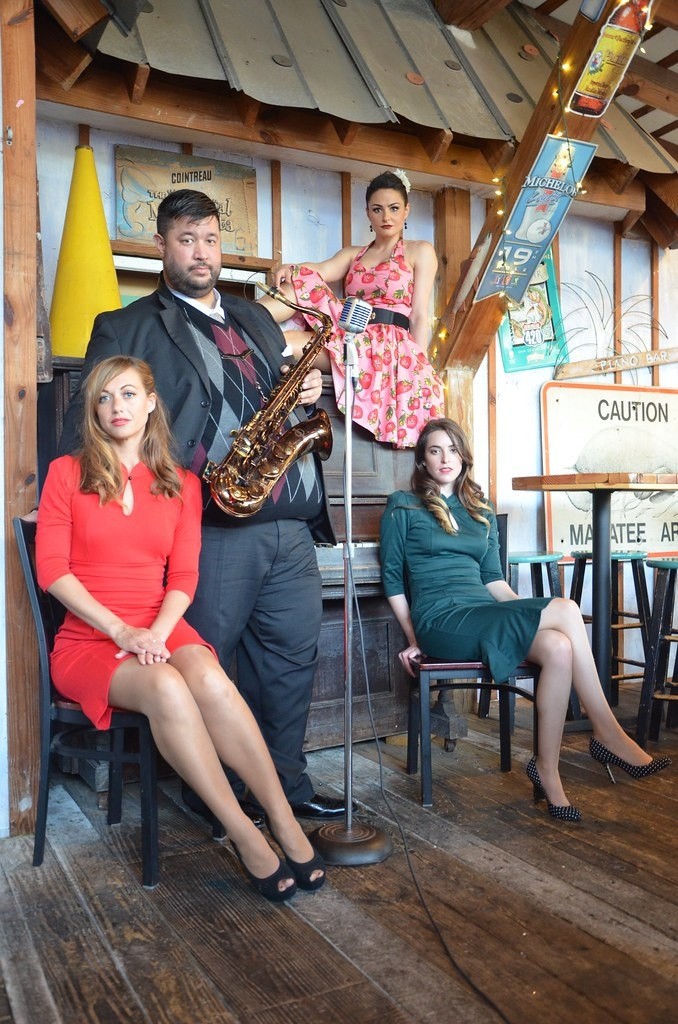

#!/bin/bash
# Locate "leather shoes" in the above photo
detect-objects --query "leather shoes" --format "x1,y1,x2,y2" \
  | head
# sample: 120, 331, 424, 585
236, 799, 262, 823
292, 793, 358, 820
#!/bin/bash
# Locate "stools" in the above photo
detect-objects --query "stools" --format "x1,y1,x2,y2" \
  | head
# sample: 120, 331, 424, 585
633, 555, 678, 753
508, 550, 566, 602
570, 548, 653, 715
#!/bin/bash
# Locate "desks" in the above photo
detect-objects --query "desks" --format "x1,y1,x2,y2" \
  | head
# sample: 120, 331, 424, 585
511, 472, 678, 737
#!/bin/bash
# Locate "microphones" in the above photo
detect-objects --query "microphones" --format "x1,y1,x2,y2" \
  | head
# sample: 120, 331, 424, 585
337, 296, 373, 390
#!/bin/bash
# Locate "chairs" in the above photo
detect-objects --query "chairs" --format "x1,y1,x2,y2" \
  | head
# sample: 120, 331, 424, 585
12, 516, 227, 890
406, 515, 541, 807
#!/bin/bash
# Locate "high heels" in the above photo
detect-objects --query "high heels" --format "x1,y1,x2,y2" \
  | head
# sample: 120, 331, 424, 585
526, 755, 582, 821
266, 816, 325, 892
230, 831, 296, 902
588, 736, 672, 783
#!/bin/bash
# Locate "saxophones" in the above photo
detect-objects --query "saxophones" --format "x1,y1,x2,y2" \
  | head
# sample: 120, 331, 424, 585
201, 279, 339, 520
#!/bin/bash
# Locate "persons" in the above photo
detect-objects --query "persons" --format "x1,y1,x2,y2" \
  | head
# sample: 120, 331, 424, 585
380, 417, 672, 821
257, 168, 446, 450
35, 355, 327, 901
23, 189, 359, 830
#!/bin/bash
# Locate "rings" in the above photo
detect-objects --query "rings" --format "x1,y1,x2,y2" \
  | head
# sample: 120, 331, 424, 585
153, 640, 157, 643
